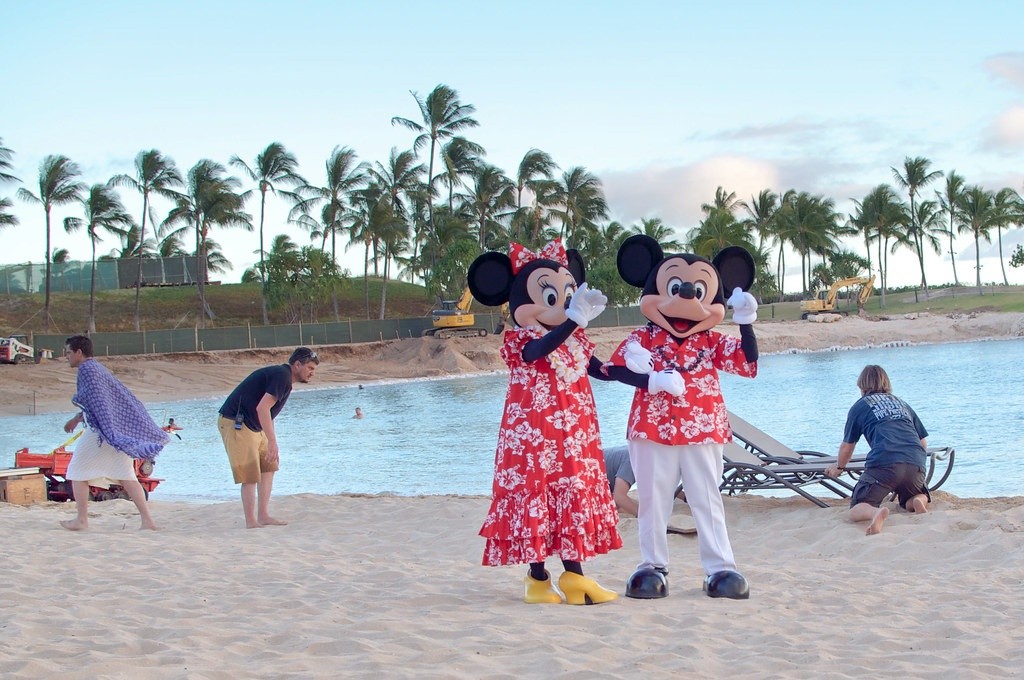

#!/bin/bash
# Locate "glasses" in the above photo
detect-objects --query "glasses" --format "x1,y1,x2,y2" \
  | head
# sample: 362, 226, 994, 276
301, 352, 317, 357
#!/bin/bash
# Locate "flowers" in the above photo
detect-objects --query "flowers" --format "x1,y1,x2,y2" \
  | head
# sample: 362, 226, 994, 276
512, 324, 587, 383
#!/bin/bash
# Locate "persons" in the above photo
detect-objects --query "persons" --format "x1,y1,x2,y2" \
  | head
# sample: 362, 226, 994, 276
217, 347, 319, 529
602, 445, 684, 517
168, 418, 176, 428
59, 336, 171, 530
352, 407, 363, 419
825, 365, 931, 535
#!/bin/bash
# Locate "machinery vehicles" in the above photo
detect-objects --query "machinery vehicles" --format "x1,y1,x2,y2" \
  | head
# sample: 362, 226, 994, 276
0, 335, 35, 365
13, 416, 183, 504
799, 274, 878, 320
420, 283, 512, 340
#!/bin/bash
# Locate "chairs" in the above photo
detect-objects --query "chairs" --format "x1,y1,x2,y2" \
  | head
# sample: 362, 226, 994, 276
672, 408, 954, 510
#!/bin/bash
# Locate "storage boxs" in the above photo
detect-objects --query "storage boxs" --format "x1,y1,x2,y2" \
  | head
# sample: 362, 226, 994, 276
0, 477, 48, 507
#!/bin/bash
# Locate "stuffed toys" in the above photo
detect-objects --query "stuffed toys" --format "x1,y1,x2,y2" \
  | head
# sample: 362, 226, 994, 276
600, 235, 759, 600
467, 238, 656, 605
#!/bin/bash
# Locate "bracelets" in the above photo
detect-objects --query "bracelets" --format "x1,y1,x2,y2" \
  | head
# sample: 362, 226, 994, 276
836, 463, 845, 469
